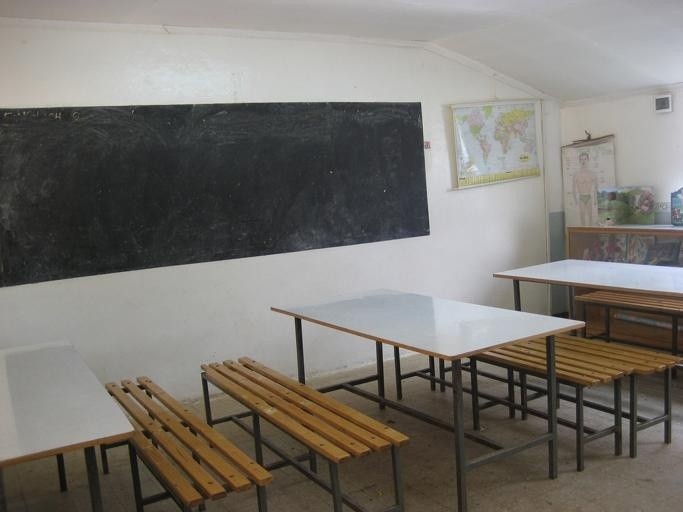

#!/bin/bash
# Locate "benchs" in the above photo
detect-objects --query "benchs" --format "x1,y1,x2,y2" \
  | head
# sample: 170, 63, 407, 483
200, 355, 411, 512
440, 334, 683, 473
573, 290, 683, 380
100, 376, 273, 511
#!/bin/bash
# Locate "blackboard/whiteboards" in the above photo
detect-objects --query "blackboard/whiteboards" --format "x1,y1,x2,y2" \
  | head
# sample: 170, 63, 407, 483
0, 102, 430, 288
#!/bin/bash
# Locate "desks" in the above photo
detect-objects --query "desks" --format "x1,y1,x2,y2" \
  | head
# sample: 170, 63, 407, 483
493, 258, 682, 313
271, 292, 587, 512
0, 340, 136, 512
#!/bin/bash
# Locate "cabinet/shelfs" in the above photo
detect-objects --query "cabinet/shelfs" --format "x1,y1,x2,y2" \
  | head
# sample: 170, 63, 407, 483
563, 222, 683, 371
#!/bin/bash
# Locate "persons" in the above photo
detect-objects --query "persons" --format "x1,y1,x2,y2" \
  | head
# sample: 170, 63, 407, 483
570, 153, 600, 229
